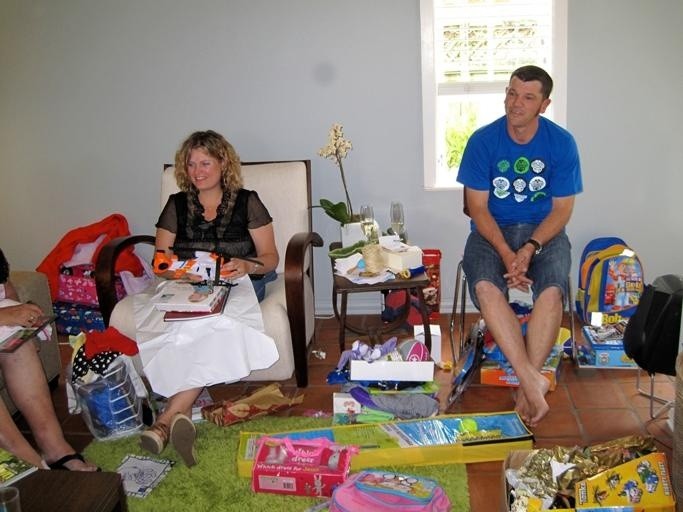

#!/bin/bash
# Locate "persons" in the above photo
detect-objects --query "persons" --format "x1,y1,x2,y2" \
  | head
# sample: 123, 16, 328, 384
456, 66, 584, 428
0, 248, 101, 472
139, 129, 280, 467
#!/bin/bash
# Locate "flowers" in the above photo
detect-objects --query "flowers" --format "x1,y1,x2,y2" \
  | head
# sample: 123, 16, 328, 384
313, 118, 354, 225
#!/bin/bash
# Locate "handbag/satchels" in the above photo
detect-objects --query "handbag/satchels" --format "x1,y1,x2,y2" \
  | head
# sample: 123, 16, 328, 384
66, 353, 149, 443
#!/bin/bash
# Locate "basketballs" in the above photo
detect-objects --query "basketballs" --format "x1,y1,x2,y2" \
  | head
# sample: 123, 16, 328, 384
396, 338, 428, 361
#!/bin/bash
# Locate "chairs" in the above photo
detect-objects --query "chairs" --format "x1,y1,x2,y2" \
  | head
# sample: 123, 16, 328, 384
447, 232, 580, 381
90, 159, 325, 388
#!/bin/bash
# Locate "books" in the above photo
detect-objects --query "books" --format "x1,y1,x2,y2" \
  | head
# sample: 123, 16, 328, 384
154, 267, 232, 323
334, 256, 394, 285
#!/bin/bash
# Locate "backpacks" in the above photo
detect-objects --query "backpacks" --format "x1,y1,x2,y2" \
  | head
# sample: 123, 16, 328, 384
574, 235, 648, 325
330, 469, 451, 512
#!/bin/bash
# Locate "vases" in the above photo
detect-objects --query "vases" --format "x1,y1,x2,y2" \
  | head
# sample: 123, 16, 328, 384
339, 220, 364, 249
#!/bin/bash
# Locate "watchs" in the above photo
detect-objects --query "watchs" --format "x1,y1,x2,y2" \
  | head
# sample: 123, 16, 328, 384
527, 238, 543, 254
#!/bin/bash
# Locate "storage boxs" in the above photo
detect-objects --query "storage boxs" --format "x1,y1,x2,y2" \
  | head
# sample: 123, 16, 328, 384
413, 323, 442, 364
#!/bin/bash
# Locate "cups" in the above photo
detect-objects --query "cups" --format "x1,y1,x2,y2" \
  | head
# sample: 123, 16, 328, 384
390, 201, 405, 235
360, 205, 374, 242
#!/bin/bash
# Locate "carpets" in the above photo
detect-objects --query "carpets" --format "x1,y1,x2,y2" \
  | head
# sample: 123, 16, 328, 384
61, 415, 472, 512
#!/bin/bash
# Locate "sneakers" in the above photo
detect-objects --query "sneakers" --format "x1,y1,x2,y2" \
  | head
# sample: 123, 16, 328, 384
138, 412, 201, 469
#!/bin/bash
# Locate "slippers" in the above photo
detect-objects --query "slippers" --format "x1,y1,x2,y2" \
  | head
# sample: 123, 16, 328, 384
44, 453, 101, 473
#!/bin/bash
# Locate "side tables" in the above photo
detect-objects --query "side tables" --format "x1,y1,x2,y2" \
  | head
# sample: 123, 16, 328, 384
331, 265, 433, 361
